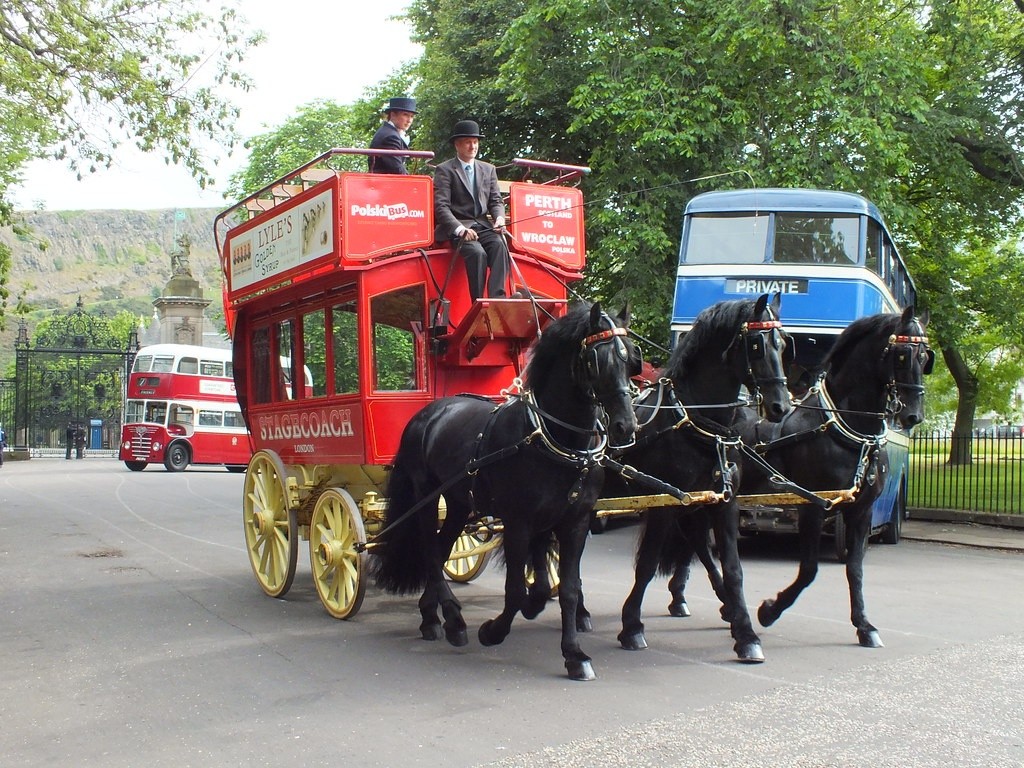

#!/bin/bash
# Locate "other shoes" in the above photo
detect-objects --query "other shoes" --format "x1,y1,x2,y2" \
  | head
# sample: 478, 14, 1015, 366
510, 292, 524, 299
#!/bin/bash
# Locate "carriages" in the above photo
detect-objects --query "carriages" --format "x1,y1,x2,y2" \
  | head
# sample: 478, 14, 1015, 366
213, 148, 931, 681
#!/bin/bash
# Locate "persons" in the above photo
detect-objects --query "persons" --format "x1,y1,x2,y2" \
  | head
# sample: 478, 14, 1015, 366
434, 120, 507, 305
0, 428, 8, 468
367, 98, 419, 175
65, 424, 84, 459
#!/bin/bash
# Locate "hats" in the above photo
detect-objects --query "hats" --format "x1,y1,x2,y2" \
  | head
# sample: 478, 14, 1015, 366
383, 98, 420, 115
448, 120, 485, 143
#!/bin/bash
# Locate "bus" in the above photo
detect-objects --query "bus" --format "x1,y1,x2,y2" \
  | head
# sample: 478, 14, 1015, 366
118, 344, 315, 473
669, 188, 936, 564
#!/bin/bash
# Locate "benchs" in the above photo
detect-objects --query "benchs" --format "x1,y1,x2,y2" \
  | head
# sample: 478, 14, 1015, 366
246, 198, 275, 220
299, 167, 533, 224
272, 184, 313, 206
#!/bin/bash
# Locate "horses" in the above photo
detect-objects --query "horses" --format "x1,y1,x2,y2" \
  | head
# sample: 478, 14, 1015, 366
521, 289, 794, 664
735, 305, 930, 649
373, 302, 644, 682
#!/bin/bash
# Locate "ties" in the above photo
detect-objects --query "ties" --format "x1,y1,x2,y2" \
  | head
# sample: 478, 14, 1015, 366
465, 164, 472, 181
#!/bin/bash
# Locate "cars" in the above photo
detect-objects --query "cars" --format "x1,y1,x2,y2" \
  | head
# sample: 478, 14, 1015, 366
997, 425, 1024, 438
